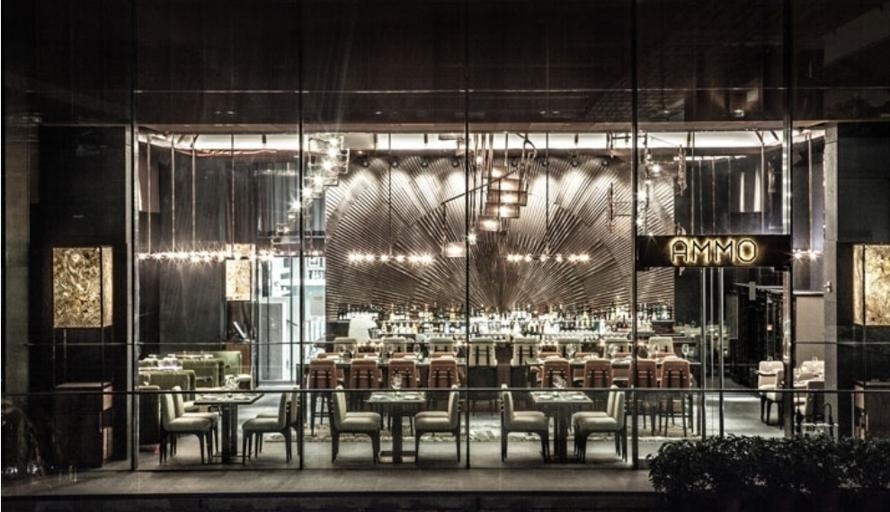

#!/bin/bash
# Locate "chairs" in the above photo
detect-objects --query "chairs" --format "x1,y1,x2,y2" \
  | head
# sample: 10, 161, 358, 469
156, 384, 304, 467
496, 381, 632, 466
137, 312, 824, 442
325, 383, 466, 465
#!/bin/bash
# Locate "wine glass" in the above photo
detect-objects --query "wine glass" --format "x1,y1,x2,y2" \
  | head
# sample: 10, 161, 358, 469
335, 344, 690, 364
391, 373, 401, 399
552, 376, 566, 400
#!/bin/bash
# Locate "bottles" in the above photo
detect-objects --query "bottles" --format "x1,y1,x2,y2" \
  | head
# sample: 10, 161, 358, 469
337, 300, 670, 342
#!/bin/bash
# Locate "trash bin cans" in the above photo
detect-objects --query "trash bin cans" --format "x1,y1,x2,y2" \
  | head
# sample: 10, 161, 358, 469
851, 380, 890, 441
54, 381, 114, 468
